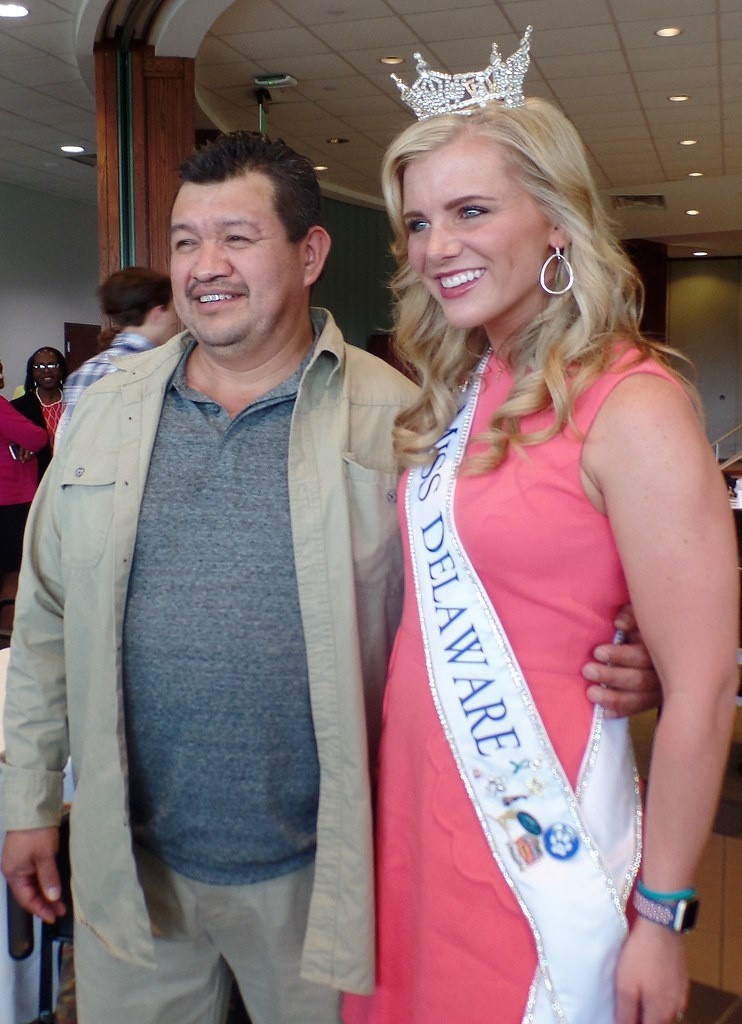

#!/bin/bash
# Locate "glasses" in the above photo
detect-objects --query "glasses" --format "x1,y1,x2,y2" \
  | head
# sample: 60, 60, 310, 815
32, 362, 60, 370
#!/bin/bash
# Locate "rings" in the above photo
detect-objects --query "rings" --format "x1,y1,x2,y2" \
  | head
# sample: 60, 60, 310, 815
29, 452, 35, 455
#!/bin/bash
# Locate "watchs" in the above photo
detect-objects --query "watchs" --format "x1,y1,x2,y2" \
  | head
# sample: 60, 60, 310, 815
630, 890, 701, 933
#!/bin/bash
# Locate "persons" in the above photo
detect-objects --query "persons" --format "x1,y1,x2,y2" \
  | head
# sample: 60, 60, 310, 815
0, 266, 176, 642
333, 25, 742, 1024
0, 127, 660, 1024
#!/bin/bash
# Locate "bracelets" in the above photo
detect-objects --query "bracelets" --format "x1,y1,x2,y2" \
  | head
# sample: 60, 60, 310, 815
636, 880, 696, 900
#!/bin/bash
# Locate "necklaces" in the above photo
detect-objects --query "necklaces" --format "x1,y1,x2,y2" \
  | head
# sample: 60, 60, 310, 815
36, 387, 63, 408
